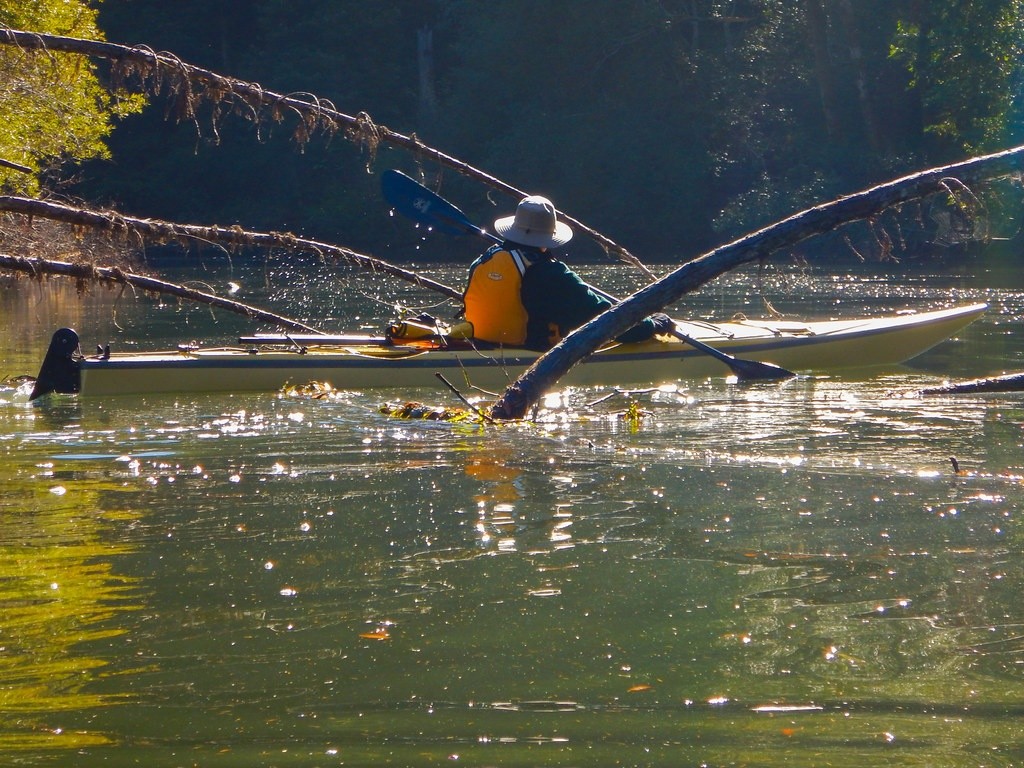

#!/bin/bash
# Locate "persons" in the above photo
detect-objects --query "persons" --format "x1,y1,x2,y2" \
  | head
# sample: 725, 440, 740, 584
462, 196, 676, 352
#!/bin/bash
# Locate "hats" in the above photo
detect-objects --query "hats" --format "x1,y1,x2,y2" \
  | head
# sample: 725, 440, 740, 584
494, 195, 573, 248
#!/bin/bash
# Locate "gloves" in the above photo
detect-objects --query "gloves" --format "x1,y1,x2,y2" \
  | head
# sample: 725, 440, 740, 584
652, 313, 676, 338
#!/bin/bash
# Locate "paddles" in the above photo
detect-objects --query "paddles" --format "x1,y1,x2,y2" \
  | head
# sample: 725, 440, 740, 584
379, 166, 797, 379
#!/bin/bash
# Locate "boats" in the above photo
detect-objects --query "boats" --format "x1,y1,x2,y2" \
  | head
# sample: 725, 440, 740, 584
72, 301, 991, 401
